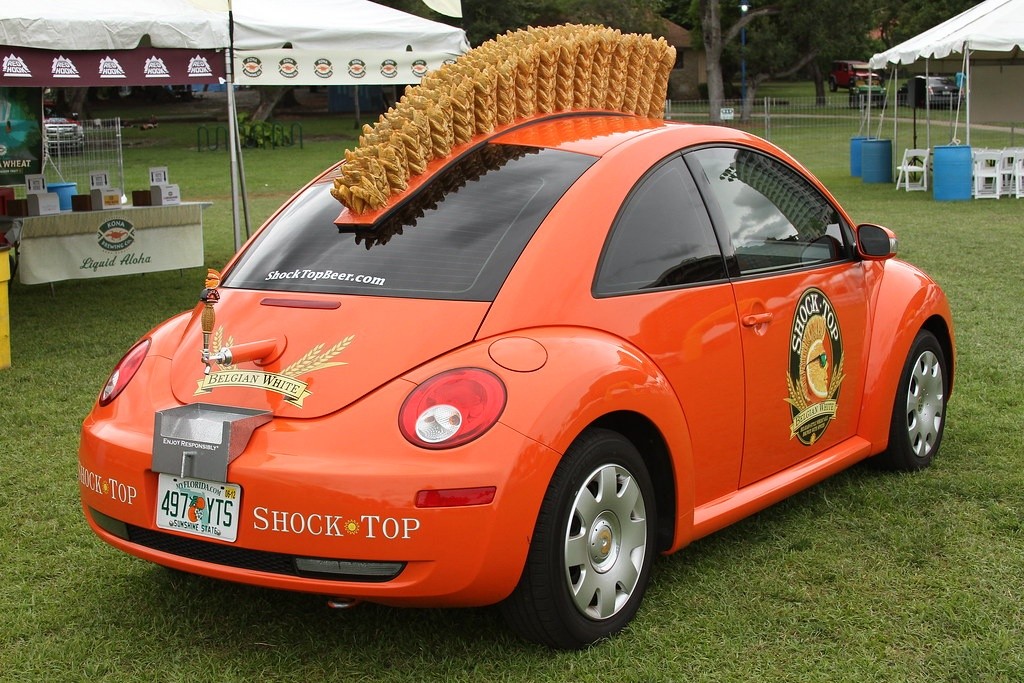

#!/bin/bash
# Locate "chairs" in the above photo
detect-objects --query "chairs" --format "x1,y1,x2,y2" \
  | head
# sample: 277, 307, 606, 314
895, 143, 1024, 200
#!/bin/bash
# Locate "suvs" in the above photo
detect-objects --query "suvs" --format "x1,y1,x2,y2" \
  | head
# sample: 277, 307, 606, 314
828, 59, 881, 94
36, 99, 84, 154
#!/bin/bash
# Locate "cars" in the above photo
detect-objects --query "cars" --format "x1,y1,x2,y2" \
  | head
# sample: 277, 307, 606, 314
74, 22, 958, 653
896, 75, 961, 109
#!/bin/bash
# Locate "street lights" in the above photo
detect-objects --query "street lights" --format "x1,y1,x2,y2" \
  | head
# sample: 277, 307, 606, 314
738, 1, 752, 115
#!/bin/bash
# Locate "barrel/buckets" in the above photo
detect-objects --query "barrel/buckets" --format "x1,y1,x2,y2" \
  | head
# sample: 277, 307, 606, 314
849, 136, 877, 178
932, 145, 971, 201
861, 139, 892, 183
